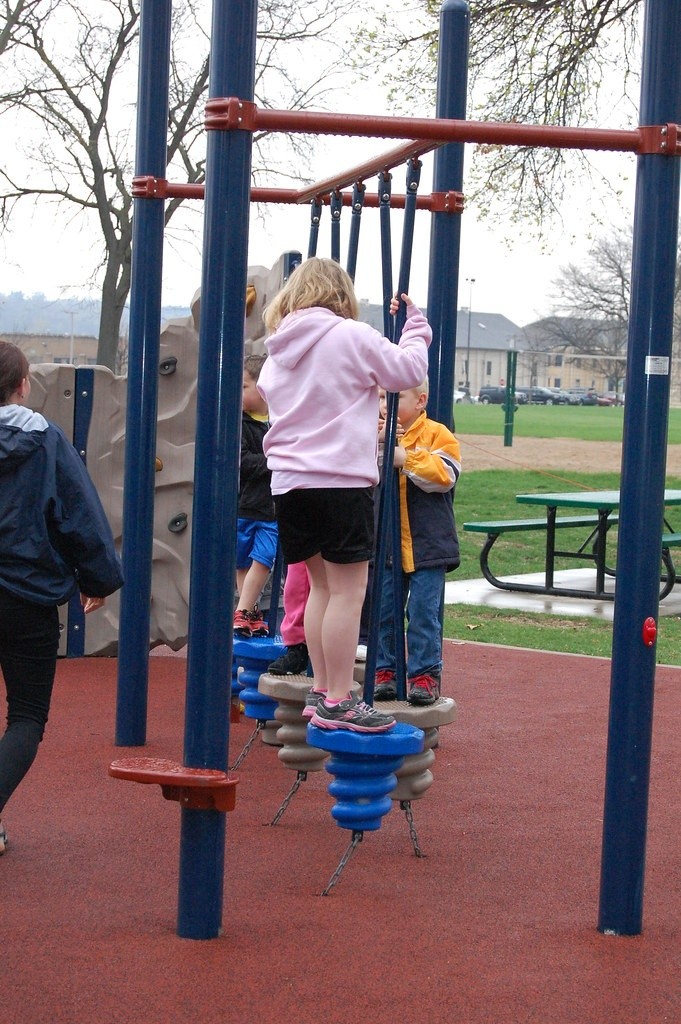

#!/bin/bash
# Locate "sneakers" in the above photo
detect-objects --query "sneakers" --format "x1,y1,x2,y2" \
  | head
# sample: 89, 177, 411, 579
311, 691, 396, 733
268, 644, 310, 676
232, 610, 252, 638
373, 673, 396, 700
301, 687, 326, 722
407, 674, 439, 704
250, 611, 269, 637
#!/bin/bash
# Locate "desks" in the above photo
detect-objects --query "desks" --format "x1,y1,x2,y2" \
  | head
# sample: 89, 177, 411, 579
462, 490, 681, 602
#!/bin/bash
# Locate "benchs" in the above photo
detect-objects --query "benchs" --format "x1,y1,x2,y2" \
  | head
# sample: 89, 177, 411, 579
662, 532, 681, 548
462, 515, 618, 534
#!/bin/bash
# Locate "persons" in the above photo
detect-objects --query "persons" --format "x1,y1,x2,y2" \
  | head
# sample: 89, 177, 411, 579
230, 354, 465, 708
0, 338, 124, 857
253, 256, 432, 734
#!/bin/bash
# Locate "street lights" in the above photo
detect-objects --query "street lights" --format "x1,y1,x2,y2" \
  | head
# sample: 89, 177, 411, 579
465, 278, 476, 388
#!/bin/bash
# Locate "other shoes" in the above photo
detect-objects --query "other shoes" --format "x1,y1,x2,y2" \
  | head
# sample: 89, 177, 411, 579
355, 645, 367, 662
0, 831, 8, 856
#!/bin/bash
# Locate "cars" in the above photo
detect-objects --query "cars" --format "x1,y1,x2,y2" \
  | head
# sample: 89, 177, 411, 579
515, 386, 624, 406
478, 385, 528, 404
453, 390, 466, 404
